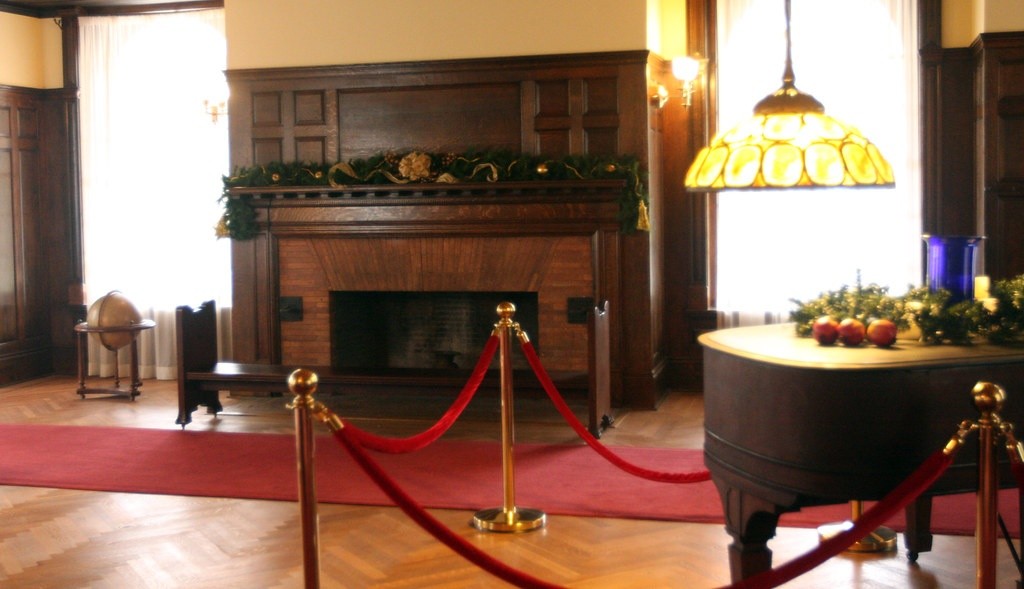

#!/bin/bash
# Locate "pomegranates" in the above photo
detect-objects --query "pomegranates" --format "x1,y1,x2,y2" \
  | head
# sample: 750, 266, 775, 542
814, 316, 898, 347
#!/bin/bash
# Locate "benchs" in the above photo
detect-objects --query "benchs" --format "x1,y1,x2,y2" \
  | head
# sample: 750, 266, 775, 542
177, 299, 618, 431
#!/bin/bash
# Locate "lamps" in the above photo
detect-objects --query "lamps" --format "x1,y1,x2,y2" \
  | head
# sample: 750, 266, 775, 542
201, 96, 228, 124
650, 52, 708, 111
683, 0, 898, 196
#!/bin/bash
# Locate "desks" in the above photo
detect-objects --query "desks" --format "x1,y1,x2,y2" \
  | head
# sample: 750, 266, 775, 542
695, 320, 1024, 589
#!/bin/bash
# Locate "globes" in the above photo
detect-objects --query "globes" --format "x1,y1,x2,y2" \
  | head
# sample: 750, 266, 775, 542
73, 289, 155, 401
